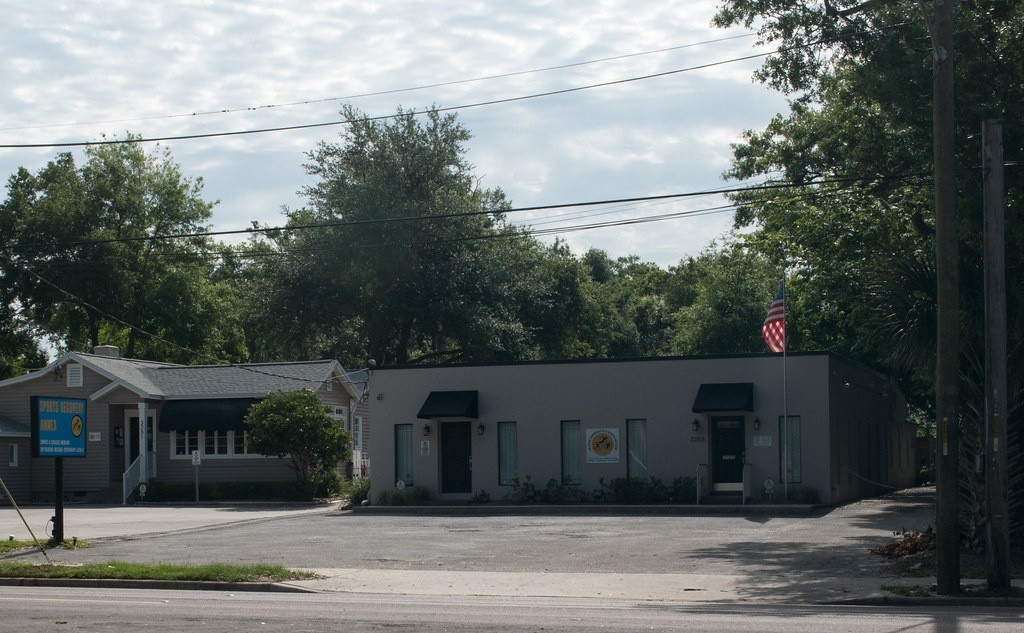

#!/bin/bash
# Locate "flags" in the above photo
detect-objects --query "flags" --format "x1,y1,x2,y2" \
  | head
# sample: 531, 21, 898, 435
762, 284, 785, 352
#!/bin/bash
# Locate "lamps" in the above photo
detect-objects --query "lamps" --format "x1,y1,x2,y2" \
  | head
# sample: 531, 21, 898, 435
478, 423, 484, 434
377, 392, 382, 400
692, 418, 699, 431
753, 416, 760, 430
422, 424, 430, 436
